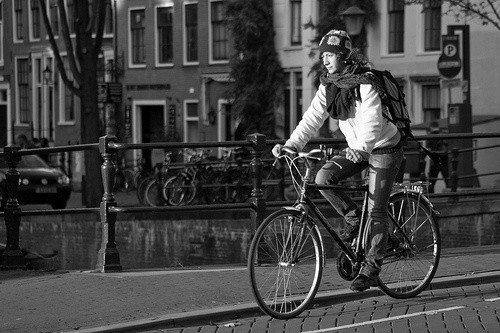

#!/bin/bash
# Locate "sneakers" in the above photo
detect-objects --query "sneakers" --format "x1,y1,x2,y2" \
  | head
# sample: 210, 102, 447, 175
350, 274, 379, 290
338, 221, 367, 242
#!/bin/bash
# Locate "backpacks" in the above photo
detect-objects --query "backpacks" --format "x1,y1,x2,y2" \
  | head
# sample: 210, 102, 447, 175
351, 65, 414, 145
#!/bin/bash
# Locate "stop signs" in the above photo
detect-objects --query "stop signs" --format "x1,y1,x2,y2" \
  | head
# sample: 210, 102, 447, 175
436, 54, 462, 78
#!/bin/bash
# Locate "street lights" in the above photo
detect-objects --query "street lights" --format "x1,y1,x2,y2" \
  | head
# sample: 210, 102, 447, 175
340, 5, 366, 46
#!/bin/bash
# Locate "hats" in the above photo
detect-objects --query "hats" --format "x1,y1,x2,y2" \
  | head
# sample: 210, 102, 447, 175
319, 29, 352, 60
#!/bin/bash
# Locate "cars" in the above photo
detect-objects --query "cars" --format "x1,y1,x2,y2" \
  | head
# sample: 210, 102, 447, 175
0, 149, 73, 210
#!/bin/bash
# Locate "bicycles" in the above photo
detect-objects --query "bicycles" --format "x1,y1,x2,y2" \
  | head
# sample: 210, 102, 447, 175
246, 144, 443, 320
112, 147, 276, 206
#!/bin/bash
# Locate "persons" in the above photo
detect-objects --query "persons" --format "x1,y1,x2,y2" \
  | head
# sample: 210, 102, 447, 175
424, 119, 450, 192
271, 28, 412, 291
15, 134, 34, 149
38, 137, 49, 163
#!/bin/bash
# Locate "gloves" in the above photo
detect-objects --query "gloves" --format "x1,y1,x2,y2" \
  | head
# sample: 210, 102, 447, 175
272, 144, 298, 158
343, 147, 369, 163
